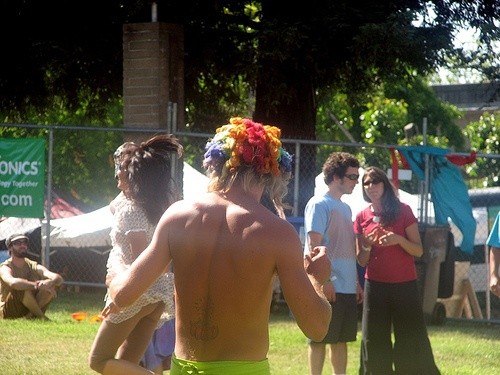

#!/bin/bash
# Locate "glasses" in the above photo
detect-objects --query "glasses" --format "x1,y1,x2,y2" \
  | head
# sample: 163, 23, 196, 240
11, 240, 29, 247
344, 173, 360, 180
363, 178, 382, 186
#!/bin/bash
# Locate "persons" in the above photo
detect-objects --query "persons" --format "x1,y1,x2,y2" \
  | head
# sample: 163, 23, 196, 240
0, 234, 64, 321
89, 117, 500, 375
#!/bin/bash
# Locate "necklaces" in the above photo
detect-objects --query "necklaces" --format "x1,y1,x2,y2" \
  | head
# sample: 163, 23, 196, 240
372, 216, 380, 224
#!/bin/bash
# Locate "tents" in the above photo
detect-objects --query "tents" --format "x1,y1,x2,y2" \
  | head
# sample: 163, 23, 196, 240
0, 162, 213, 266
314, 165, 479, 226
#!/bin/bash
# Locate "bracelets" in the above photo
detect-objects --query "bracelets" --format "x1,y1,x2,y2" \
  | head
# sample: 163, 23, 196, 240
361, 242, 372, 251
324, 278, 332, 283
34, 281, 38, 291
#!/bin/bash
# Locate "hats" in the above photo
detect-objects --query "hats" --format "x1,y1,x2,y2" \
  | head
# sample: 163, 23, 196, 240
4, 233, 30, 248
